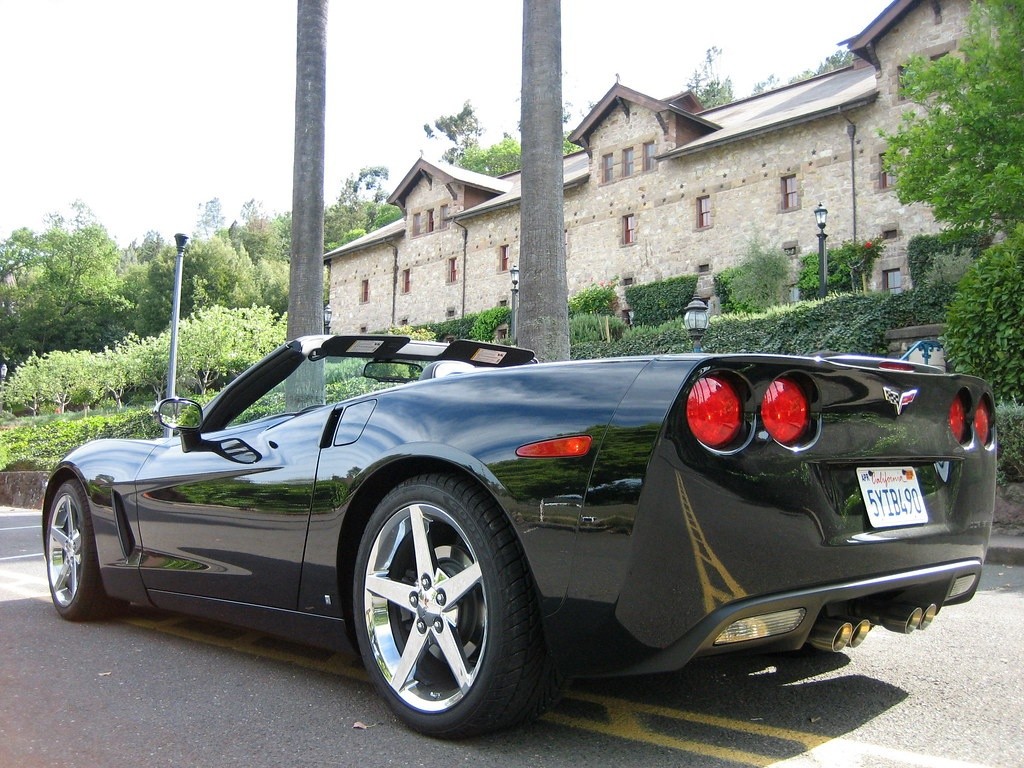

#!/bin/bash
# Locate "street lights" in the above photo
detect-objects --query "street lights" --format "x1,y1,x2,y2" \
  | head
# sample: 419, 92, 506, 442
164, 232, 191, 441
814, 202, 831, 301
0, 363, 8, 392
509, 263, 520, 343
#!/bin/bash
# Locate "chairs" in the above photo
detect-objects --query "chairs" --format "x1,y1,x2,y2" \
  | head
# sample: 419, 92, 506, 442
418, 359, 475, 381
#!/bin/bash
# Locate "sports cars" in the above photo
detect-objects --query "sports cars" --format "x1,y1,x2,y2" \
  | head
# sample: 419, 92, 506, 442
40, 331, 1001, 746
